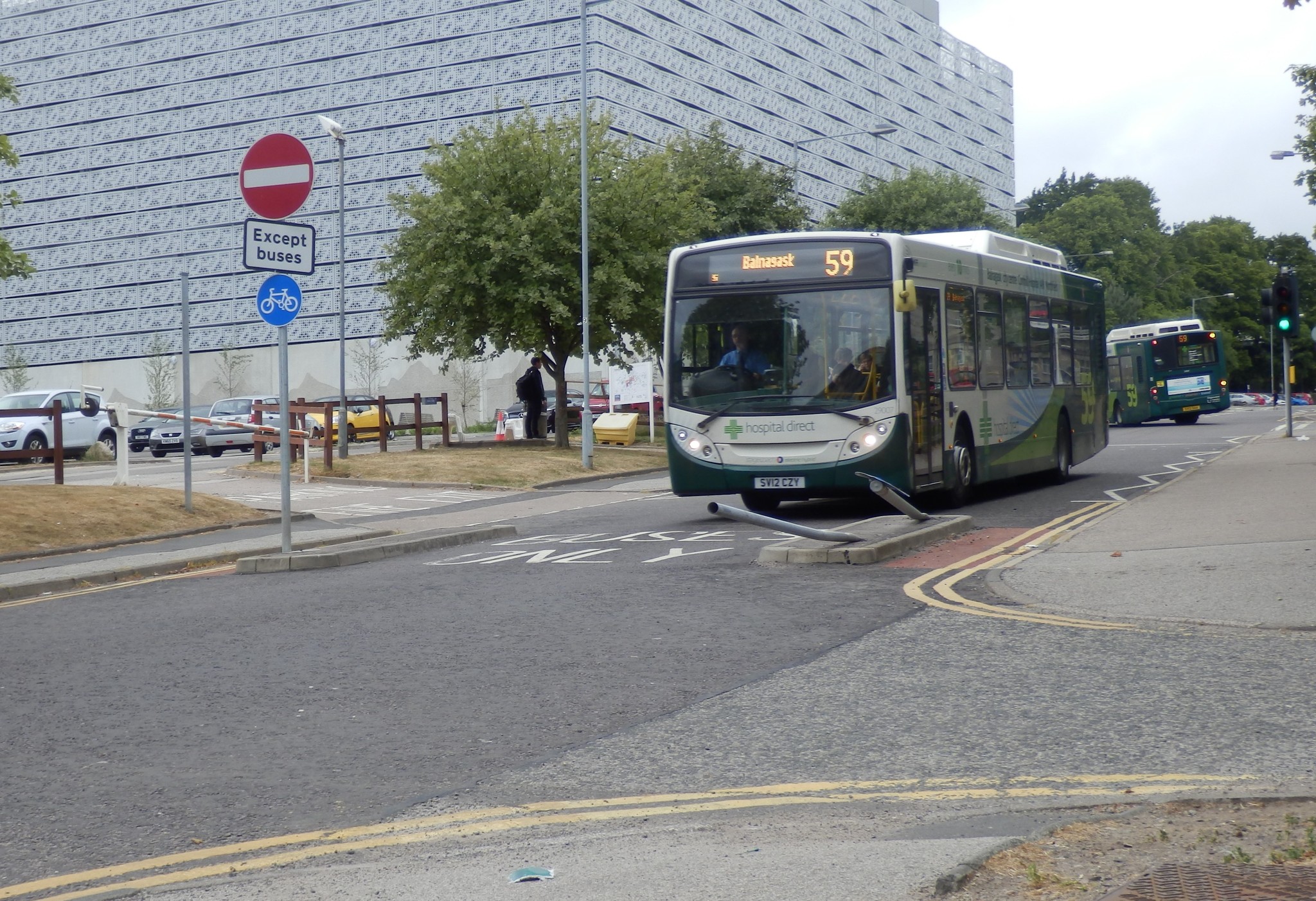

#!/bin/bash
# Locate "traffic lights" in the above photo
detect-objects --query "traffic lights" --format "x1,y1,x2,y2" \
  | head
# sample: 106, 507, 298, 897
1260, 287, 1275, 327
1271, 273, 1301, 339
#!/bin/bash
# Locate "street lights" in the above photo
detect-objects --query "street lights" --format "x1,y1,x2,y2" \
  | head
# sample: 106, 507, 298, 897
793, 124, 898, 208
1192, 292, 1235, 320
317, 114, 349, 460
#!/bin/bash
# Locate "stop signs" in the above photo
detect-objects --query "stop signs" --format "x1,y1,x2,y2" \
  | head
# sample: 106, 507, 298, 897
240, 134, 313, 222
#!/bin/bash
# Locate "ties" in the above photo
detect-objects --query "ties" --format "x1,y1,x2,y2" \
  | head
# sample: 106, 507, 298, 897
737, 356, 745, 368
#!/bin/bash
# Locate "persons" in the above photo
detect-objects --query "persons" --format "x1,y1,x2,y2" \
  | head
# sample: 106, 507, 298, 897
809, 338, 1080, 400
516, 357, 547, 439
719, 327, 768, 385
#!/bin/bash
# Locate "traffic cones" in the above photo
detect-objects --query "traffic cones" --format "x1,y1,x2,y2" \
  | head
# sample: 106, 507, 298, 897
496, 412, 505, 441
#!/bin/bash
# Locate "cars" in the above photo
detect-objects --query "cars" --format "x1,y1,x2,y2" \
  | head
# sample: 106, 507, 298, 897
205, 396, 320, 458
1230, 391, 1314, 406
306, 394, 395, 443
127, 407, 181, 453
496, 388, 584, 435
149, 404, 214, 458
579, 380, 664, 421
0, 388, 118, 465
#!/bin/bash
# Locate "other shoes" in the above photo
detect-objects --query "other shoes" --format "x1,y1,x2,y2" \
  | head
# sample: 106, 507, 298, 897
526, 435, 533, 440
533, 435, 546, 439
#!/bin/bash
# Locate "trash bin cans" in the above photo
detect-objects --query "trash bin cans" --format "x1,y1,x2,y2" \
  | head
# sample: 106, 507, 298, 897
592, 412, 639, 446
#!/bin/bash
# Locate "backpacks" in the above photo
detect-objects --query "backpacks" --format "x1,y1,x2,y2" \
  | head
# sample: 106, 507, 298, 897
516, 369, 535, 401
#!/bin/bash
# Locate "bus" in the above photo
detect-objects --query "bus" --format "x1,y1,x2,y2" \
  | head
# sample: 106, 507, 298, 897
1105, 319, 1231, 429
661, 229, 1112, 514
